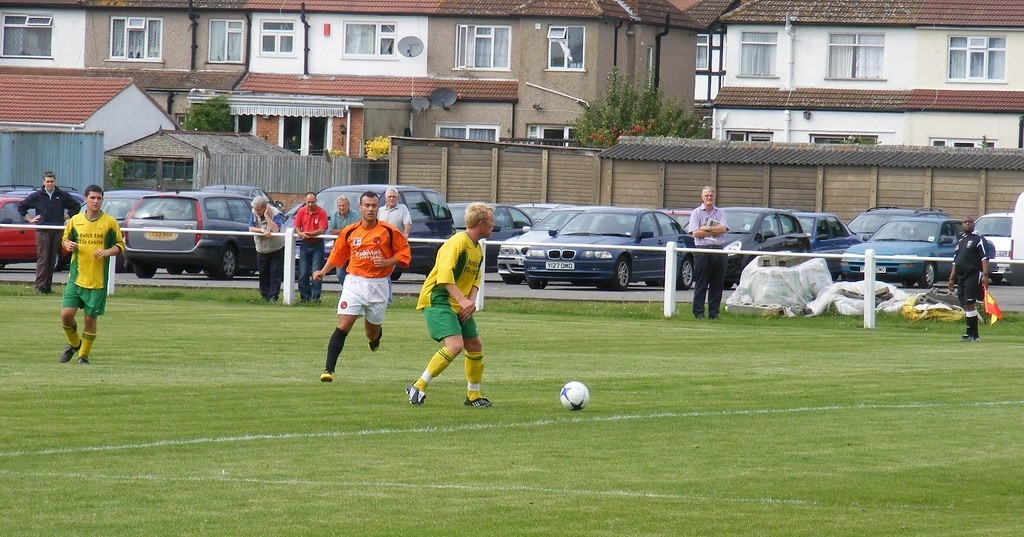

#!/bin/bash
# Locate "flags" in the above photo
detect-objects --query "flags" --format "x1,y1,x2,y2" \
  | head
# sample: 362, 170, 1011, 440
984, 291, 1003, 325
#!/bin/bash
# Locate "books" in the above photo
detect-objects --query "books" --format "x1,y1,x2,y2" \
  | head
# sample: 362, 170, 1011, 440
704, 218, 720, 242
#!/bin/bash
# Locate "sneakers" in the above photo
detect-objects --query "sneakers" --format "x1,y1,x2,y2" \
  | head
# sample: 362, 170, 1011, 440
59, 340, 82, 363
404, 384, 426, 406
465, 396, 494, 408
78, 355, 89, 364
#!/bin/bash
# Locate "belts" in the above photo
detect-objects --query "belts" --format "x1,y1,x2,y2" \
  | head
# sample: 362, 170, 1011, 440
697, 245, 724, 248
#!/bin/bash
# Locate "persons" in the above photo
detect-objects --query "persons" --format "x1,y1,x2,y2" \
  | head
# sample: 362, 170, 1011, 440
292, 191, 329, 307
59, 184, 125, 365
374, 187, 412, 281
948, 215, 990, 342
314, 190, 413, 385
688, 185, 730, 320
328, 196, 361, 288
18, 170, 81, 294
248, 195, 286, 305
403, 202, 497, 409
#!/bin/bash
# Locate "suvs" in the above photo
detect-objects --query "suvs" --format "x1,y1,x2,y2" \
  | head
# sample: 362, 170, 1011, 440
846, 206, 951, 242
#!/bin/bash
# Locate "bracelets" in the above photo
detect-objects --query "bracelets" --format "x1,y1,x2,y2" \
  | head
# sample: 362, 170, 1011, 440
949, 278, 953, 280
261, 229, 264, 232
982, 273, 988, 276
706, 226, 711, 232
404, 231, 409, 237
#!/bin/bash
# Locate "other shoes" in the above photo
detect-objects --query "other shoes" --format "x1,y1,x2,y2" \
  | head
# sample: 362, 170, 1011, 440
35, 288, 55, 296
313, 297, 321, 303
971, 336, 981, 343
368, 327, 382, 352
320, 370, 334, 382
709, 314, 721, 320
958, 333, 971, 341
695, 312, 707, 319
299, 298, 309, 303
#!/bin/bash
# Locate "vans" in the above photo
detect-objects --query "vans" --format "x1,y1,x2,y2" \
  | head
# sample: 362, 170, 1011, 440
971, 213, 1014, 282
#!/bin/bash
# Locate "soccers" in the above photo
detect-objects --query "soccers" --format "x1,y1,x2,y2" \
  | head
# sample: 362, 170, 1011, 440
560, 381, 589, 410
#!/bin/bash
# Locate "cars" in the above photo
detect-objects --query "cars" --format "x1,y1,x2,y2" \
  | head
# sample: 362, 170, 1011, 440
838, 218, 965, 288
446, 202, 696, 289
785, 209, 861, 274
281, 184, 456, 281
658, 207, 811, 290
0, 185, 286, 281
